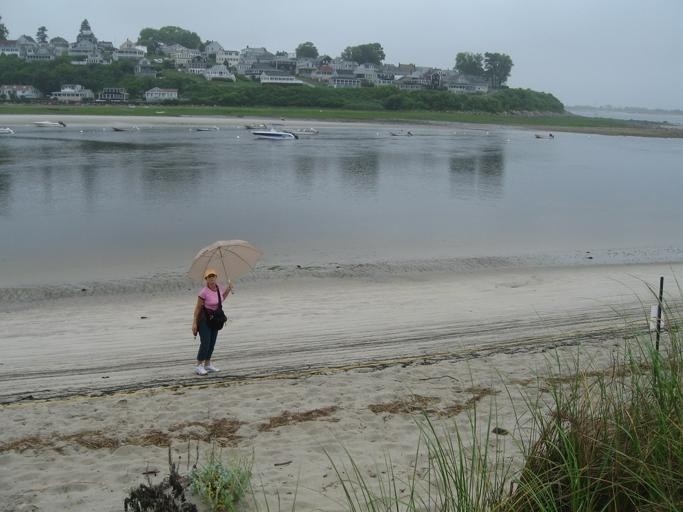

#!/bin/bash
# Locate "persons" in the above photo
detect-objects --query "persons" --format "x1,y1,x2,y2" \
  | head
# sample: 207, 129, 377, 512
191, 269, 235, 375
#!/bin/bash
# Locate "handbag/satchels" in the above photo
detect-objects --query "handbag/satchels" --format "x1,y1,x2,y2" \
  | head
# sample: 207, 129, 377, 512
204, 305, 228, 330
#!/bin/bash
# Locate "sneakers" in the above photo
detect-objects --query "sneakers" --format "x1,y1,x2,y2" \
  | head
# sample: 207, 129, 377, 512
195, 364, 207, 376
204, 364, 222, 373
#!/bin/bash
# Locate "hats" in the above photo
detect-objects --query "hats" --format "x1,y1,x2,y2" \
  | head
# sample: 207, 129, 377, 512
202, 269, 217, 279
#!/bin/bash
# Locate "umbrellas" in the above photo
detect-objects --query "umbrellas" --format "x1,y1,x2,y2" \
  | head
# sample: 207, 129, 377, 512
189, 238, 258, 296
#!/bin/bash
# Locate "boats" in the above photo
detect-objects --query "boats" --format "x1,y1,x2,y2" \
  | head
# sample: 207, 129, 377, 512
387, 127, 415, 136
111, 126, 140, 132
32, 120, 67, 128
197, 126, 220, 131
0, 127, 16, 135
245, 123, 319, 140
534, 133, 557, 139
